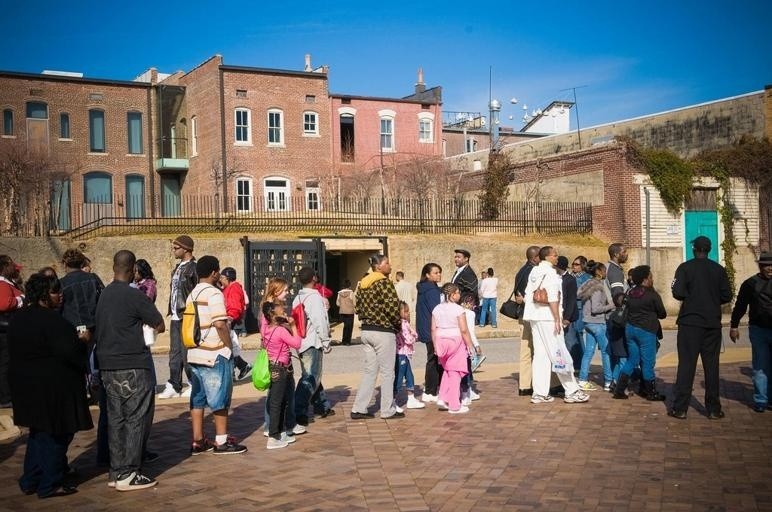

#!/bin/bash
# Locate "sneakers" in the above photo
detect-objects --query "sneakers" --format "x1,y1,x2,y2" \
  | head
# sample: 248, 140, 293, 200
238, 363, 252, 379
472, 356, 485, 371
263, 409, 335, 450
116, 471, 159, 492
143, 451, 161, 464
159, 382, 180, 399
351, 385, 480, 419
190, 437, 214, 456
212, 440, 248, 455
182, 382, 193, 397
518, 367, 666, 404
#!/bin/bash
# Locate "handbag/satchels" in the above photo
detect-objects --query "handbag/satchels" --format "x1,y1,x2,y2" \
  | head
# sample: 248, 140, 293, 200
611, 295, 630, 327
499, 299, 525, 320
532, 288, 561, 305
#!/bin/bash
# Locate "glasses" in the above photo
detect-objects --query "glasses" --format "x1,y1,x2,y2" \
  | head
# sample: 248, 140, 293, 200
173, 246, 181, 250
572, 262, 580, 266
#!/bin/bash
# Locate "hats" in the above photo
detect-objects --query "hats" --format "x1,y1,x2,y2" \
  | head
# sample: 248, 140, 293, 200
454, 249, 470, 257
754, 251, 772, 265
690, 236, 711, 251
173, 235, 194, 253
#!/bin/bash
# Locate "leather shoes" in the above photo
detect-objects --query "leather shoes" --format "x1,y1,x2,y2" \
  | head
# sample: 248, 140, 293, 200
707, 410, 725, 420
670, 409, 687, 419
38, 484, 78, 499
753, 403, 765, 413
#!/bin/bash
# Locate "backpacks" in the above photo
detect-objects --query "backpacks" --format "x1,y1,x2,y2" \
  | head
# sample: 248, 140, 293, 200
180, 285, 212, 349
252, 349, 272, 391
290, 294, 314, 338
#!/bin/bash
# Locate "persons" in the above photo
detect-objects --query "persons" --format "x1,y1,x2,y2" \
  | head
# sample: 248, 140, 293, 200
668, 237, 733, 419
730, 251, 772, 412
